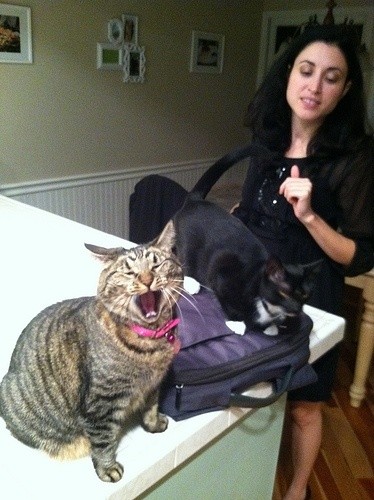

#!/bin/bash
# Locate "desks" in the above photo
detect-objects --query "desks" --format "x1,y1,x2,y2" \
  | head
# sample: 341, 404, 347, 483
1, 194, 346, 500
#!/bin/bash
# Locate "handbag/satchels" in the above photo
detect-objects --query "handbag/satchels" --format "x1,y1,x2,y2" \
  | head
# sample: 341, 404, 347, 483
161, 289, 317, 422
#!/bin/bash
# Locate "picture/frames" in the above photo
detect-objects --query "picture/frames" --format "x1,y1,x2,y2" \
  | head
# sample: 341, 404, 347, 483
122, 14, 139, 42
0, 4, 34, 64
123, 43, 146, 83
108, 17, 123, 43
189, 30, 226, 75
97, 42, 123, 71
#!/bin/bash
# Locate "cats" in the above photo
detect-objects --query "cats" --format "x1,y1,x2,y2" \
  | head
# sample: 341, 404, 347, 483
0, 219, 213, 484
170, 148, 325, 336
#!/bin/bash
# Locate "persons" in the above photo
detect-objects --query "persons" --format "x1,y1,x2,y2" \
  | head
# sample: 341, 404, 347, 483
230, 24, 374, 500
